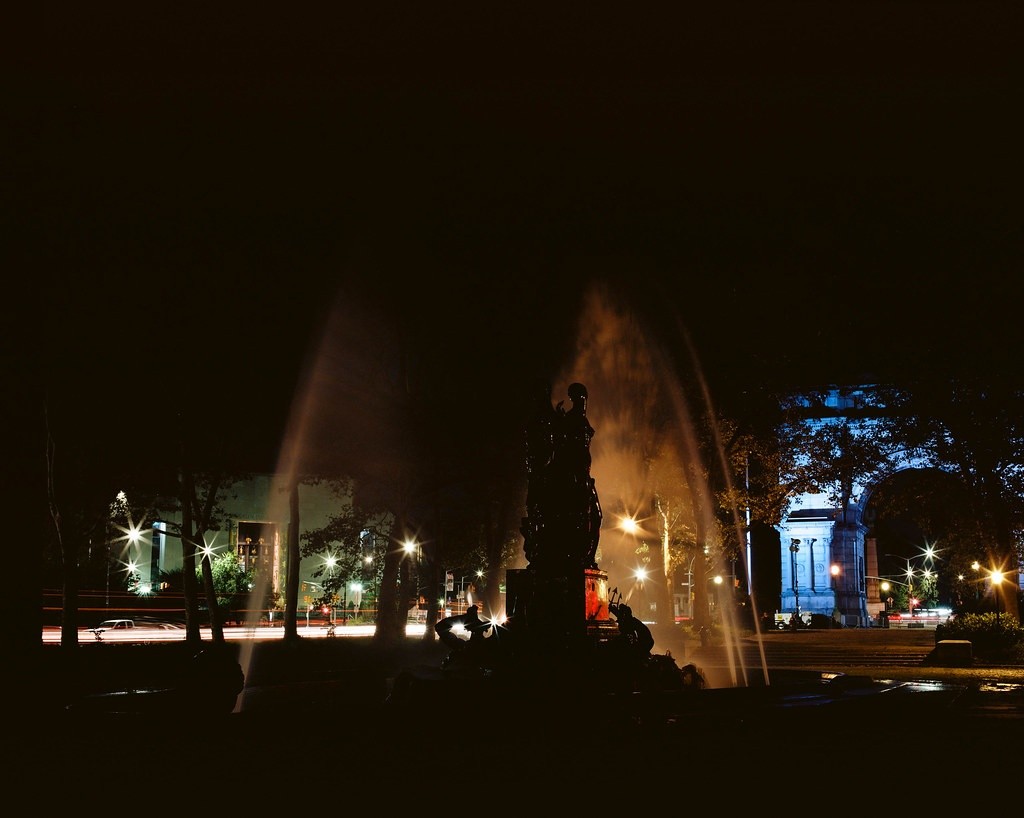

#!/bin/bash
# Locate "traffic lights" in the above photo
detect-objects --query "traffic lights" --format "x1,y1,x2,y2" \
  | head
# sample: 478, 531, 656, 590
913, 596, 916, 604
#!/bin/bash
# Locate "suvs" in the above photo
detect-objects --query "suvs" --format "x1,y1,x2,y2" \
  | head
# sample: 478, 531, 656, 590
91, 620, 134, 631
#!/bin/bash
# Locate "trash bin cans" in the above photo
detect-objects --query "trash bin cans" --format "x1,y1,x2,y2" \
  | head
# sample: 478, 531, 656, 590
880, 611, 891, 627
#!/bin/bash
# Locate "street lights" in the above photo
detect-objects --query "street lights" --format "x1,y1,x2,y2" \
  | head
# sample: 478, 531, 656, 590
991, 570, 1003, 628
106, 521, 139, 604
326, 558, 336, 621
351, 583, 362, 619
461, 571, 483, 614
635, 570, 646, 621
831, 565, 840, 610
885, 551, 933, 614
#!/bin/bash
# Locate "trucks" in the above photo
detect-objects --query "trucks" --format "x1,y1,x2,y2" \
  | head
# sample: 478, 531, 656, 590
774, 613, 791, 630
